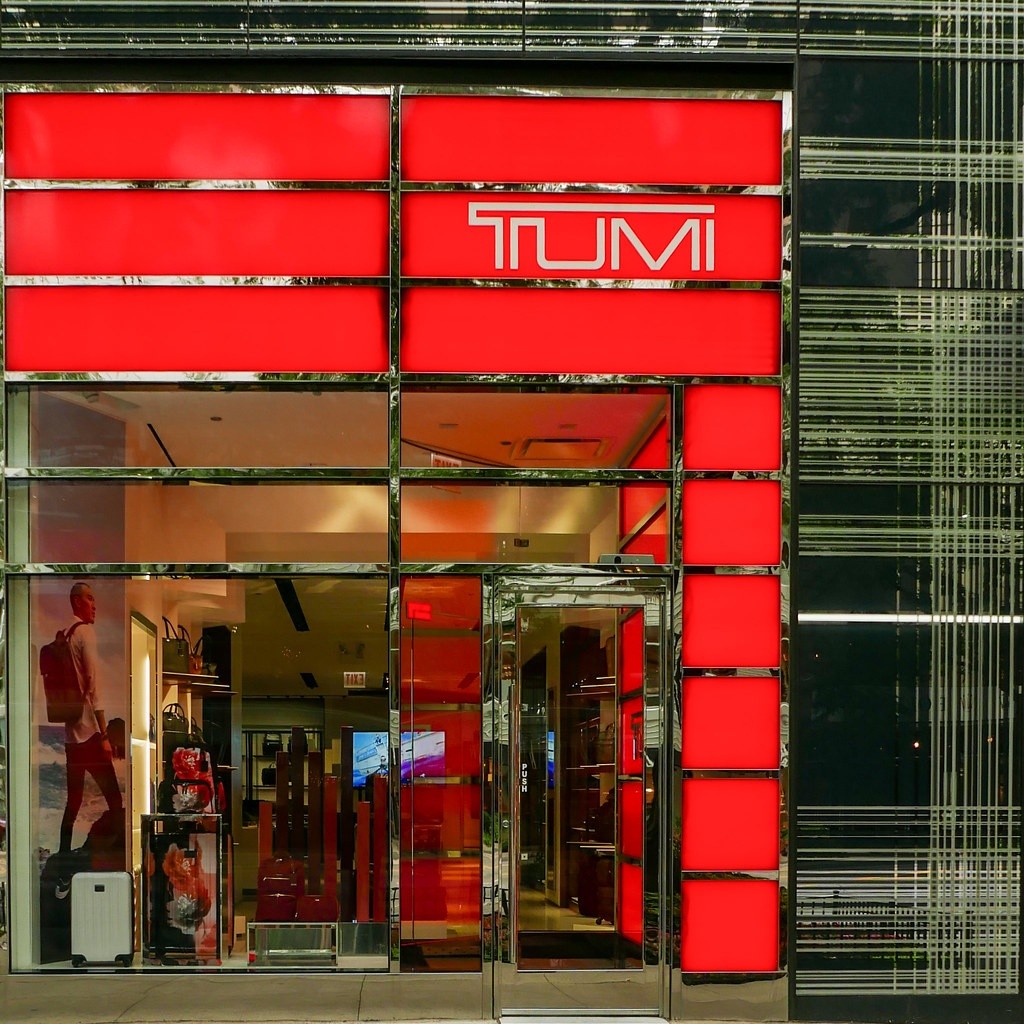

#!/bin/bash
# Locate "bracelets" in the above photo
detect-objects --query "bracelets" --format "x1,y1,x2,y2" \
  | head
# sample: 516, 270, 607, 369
100, 731, 109, 741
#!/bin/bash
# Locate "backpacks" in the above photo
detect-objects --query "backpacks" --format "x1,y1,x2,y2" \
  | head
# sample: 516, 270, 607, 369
40, 622, 88, 723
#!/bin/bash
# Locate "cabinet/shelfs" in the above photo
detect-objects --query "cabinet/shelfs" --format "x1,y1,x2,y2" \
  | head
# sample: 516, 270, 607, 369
249, 730, 323, 812
157, 620, 244, 973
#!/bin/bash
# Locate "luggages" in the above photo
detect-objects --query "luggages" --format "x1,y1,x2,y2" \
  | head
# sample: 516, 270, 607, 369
70, 871, 134, 968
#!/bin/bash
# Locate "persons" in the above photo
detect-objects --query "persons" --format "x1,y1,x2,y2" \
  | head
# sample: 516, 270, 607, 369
60, 582, 125, 854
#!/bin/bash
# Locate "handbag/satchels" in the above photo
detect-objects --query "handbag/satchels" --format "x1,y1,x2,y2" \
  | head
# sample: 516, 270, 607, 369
162, 616, 189, 673
190, 717, 209, 752
163, 703, 189, 761
178, 625, 217, 676
256, 848, 339, 923
262, 733, 283, 755
288, 737, 308, 755
262, 762, 276, 785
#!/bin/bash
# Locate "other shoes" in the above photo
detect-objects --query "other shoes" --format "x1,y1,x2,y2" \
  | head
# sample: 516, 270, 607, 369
55, 880, 69, 900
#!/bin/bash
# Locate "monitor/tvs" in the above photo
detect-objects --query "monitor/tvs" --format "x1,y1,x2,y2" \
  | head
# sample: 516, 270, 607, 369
350, 730, 446, 790
